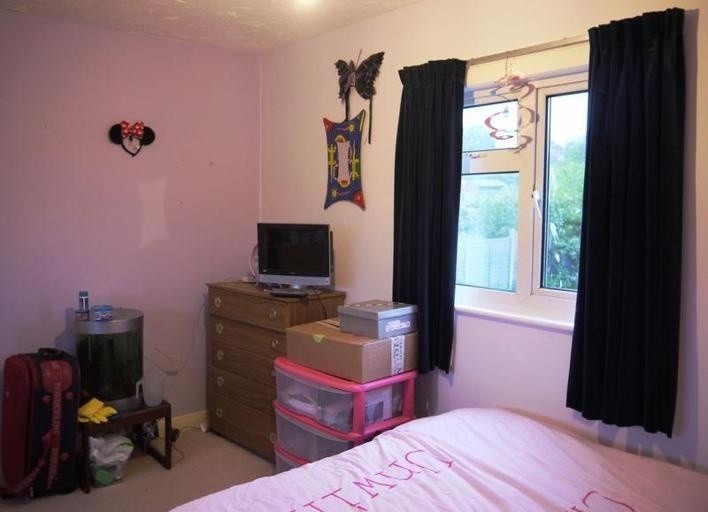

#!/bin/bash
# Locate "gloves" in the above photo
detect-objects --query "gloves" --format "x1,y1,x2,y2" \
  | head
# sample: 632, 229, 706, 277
78, 398, 118, 425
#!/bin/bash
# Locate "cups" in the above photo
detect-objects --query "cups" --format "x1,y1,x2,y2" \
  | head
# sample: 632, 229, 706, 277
78, 291, 88, 312
135, 374, 163, 408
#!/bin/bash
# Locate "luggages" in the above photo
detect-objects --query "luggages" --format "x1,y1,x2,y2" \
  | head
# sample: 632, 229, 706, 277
0, 347, 83, 501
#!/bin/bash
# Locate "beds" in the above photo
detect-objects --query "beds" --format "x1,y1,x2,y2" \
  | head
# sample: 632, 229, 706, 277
171, 406, 707, 511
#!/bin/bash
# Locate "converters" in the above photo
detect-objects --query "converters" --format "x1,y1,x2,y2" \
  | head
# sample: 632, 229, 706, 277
172, 428, 180, 442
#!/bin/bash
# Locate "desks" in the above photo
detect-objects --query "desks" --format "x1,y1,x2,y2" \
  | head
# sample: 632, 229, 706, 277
77, 399, 171, 493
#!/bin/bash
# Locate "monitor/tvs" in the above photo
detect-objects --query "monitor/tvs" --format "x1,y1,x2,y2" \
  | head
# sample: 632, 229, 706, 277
257, 223, 331, 295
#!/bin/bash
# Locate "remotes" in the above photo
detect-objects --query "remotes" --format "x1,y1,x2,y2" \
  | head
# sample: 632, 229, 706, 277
271, 289, 308, 297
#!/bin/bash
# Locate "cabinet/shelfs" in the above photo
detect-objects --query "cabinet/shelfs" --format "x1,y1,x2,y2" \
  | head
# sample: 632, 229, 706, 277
205, 281, 346, 463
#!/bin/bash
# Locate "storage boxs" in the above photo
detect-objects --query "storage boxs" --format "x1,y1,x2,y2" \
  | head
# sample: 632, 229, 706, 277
274, 301, 418, 475
